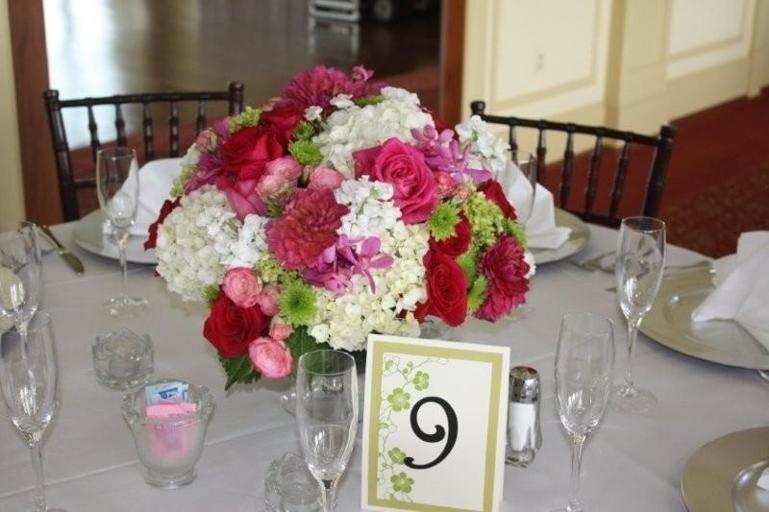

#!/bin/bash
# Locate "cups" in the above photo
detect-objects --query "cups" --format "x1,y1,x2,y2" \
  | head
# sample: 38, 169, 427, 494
91, 329, 152, 391
262, 450, 324, 512
123, 374, 215, 489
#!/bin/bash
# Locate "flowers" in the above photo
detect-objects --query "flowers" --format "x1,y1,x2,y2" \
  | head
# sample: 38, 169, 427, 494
124, 51, 544, 396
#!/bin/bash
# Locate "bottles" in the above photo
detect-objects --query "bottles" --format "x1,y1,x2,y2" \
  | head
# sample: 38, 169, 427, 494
504, 365, 545, 463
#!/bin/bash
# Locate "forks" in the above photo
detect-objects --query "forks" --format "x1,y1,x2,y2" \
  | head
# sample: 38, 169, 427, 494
565, 250, 614, 273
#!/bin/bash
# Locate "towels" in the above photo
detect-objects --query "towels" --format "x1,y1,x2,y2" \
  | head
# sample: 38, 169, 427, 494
98, 151, 187, 241
685, 221, 769, 353
488, 150, 576, 256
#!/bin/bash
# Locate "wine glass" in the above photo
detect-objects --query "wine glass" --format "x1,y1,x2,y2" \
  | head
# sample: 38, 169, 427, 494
547, 309, 618, 512
0, 308, 56, 510
0, 220, 45, 359
94, 144, 150, 317
607, 214, 667, 415
294, 348, 359, 512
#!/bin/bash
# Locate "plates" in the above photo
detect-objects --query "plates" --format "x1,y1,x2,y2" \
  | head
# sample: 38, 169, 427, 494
614, 267, 766, 374
74, 206, 157, 265
527, 205, 588, 264
0, 266, 27, 341
677, 423, 769, 511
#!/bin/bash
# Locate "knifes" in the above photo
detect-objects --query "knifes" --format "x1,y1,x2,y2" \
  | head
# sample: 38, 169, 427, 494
38, 225, 86, 279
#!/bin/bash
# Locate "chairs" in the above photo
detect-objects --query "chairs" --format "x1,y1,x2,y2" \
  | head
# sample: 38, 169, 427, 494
42, 82, 247, 226
465, 96, 681, 234
